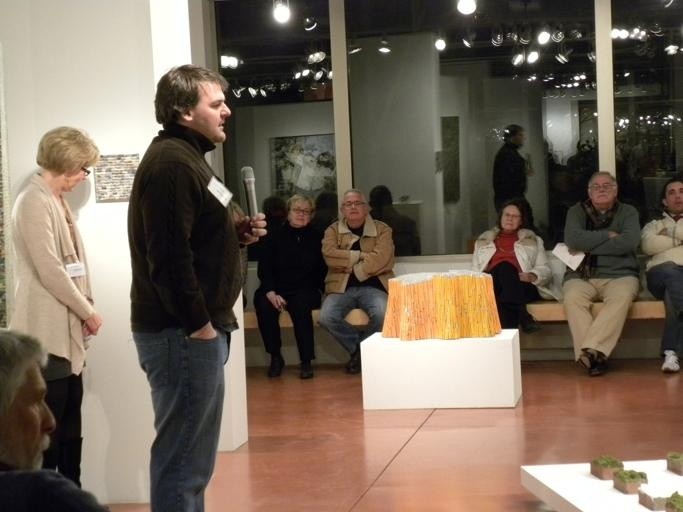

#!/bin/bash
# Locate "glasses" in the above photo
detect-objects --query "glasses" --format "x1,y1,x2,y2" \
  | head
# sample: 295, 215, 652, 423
80, 167, 90, 176
341, 200, 366, 208
289, 207, 312, 216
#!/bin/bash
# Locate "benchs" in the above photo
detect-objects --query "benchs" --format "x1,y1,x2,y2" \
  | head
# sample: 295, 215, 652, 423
243, 297, 665, 331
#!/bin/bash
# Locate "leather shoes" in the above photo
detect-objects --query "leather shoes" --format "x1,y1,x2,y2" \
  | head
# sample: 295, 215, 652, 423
575, 352, 611, 376
345, 346, 363, 377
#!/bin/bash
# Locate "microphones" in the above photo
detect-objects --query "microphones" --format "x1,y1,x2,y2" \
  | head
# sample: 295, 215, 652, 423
240, 166, 259, 224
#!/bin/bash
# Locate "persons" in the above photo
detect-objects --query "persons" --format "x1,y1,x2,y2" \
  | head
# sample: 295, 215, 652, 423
125, 65, 268, 512
248, 186, 420, 262
641, 179, 683, 374
473, 198, 552, 333
253, 193, 315, 381
319, 190, 396, 375
10, 126, 101, 487
0, 329, 108, 512
492, 125, 539, 233
557, 170, 641, 377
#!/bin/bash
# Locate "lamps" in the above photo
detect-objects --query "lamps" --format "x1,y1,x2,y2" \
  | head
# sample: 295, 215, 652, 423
455, 1, 475, 16
434, 23, 683, 100
378, 36, 392, 55
221, 0, 363, 100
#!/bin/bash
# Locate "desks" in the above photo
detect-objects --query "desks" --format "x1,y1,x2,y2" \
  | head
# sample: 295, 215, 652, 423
519, 459, 683, 511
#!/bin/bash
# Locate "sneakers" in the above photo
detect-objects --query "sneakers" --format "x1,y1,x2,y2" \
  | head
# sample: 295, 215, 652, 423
660, 349, 681, 374
299, 359, 315, 380
521, 318, 540, 334
265, 356, 285, 378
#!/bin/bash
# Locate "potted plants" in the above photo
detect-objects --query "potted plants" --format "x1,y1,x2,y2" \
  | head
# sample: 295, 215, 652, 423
667, 451, 683, 476
613, 470, 683, 512
590, 455, 624, 481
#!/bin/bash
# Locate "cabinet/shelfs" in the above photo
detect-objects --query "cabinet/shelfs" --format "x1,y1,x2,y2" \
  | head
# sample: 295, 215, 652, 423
391, 201, 426, 255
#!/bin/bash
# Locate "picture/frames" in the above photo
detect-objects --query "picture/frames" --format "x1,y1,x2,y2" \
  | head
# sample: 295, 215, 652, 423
268, 132, 338, 204
